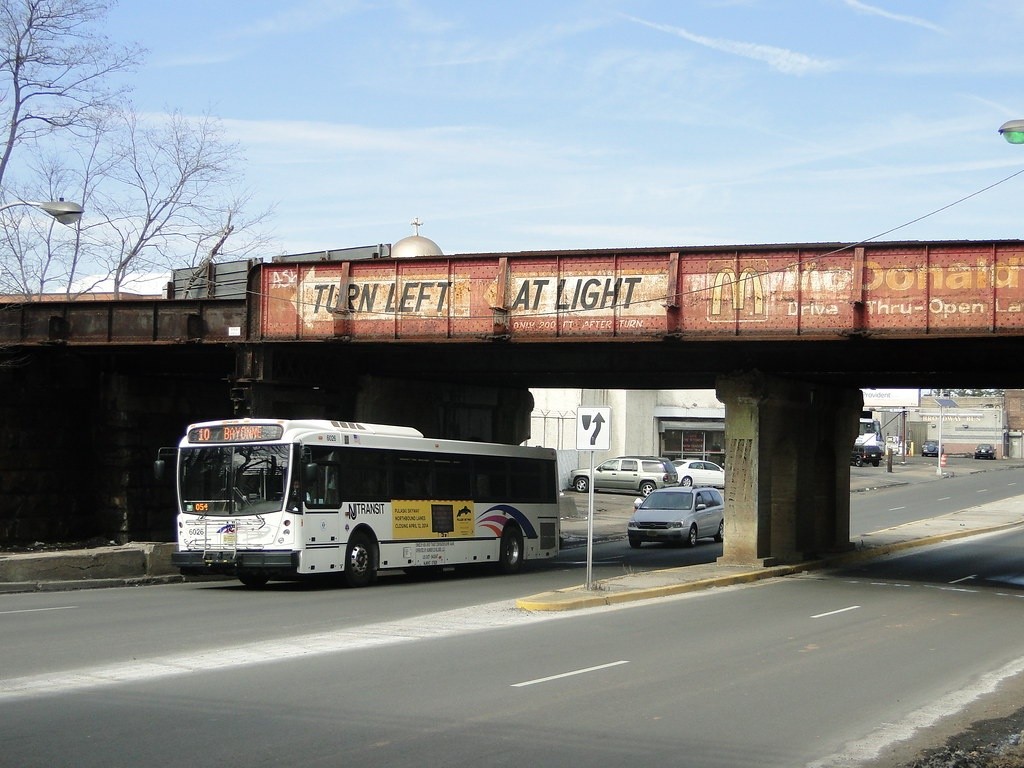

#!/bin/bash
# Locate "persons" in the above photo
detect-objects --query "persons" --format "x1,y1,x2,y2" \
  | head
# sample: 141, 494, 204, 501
293, 479, 311, 504
243, 476, 261, 499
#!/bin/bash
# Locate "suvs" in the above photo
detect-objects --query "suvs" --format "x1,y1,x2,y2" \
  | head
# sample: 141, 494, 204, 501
921, 439, 944, 457
567, 455, 680, 497
851, 444, 883, 467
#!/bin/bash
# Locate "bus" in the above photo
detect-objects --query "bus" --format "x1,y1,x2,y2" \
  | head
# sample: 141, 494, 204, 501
154, 419, 561, 590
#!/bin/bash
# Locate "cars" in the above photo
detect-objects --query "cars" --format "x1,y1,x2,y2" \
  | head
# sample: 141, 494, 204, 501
669, 458, 725, 489
627, 486, 724, 548
974, 444, 996, 460
